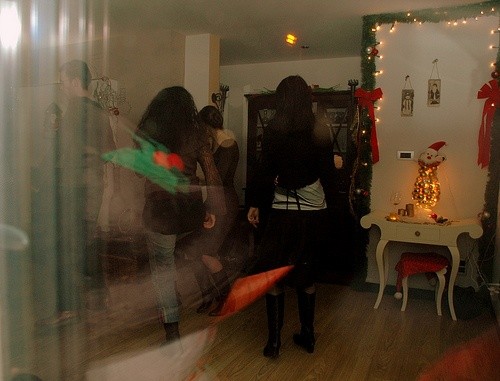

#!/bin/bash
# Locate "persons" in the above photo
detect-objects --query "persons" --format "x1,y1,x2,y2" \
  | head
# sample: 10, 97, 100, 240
185, 106, 237, 316
244, 75, 343, 357
36, 60, 111, 330
131, 86, 221, 355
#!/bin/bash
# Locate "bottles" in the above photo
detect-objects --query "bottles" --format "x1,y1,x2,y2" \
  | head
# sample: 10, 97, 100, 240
262, 110, 268, 124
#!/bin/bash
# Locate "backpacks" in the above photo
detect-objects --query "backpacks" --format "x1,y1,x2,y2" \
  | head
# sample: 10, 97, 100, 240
452, 285, 481, 320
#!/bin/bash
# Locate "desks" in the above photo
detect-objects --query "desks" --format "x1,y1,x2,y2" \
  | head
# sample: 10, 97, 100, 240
360, 210, 483, 321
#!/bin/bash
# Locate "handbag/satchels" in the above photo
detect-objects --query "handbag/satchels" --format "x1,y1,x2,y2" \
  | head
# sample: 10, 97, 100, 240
327, 167, 364, 206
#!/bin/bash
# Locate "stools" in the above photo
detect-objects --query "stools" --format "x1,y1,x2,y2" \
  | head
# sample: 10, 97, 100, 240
98, 228, 152, 285
396, 252, 448, 315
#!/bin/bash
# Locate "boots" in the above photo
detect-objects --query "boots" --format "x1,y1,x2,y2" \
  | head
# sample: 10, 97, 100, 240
292, 290, 316, 353
262, 293, 283, 359
160, 322, 182, 355
195, 266, 215, 312
209, 268, 235, 316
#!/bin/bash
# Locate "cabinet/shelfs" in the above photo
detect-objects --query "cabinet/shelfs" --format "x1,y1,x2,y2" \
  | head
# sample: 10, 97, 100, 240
244, 90, 370, 286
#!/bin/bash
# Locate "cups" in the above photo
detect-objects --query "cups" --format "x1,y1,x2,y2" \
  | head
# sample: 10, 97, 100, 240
398, 209, 404, 215
406, 204, 414, 217
